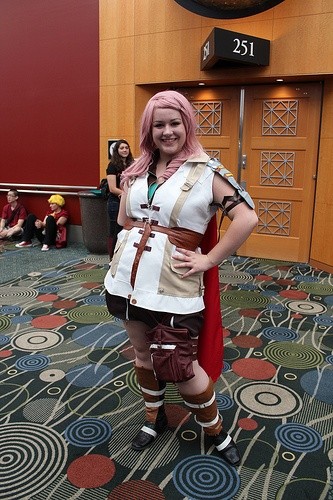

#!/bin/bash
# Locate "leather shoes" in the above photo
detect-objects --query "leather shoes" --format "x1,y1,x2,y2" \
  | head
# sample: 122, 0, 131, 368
210, 427, 241, 467
131, 416, 168, 450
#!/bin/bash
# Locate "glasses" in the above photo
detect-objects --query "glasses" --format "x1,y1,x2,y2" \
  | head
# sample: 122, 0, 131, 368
7, 195, 16, 197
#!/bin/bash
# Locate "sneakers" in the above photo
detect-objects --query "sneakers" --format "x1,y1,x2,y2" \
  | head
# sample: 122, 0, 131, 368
15, 241, 33, 247
41, 244, 50, 251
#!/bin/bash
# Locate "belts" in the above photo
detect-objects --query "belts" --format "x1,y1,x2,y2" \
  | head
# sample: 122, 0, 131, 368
124, 219, 203, 289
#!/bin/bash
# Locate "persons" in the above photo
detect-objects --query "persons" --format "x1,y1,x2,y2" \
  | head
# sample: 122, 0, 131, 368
15, 195, 69, 251
106, 140, 134, 259
0, 190, 26, 240
104, 91, 257, 465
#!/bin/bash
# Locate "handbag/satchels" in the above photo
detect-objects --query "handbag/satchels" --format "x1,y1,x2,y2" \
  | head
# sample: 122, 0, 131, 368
100, 179, 121, 202
145, 324, 195, 383
